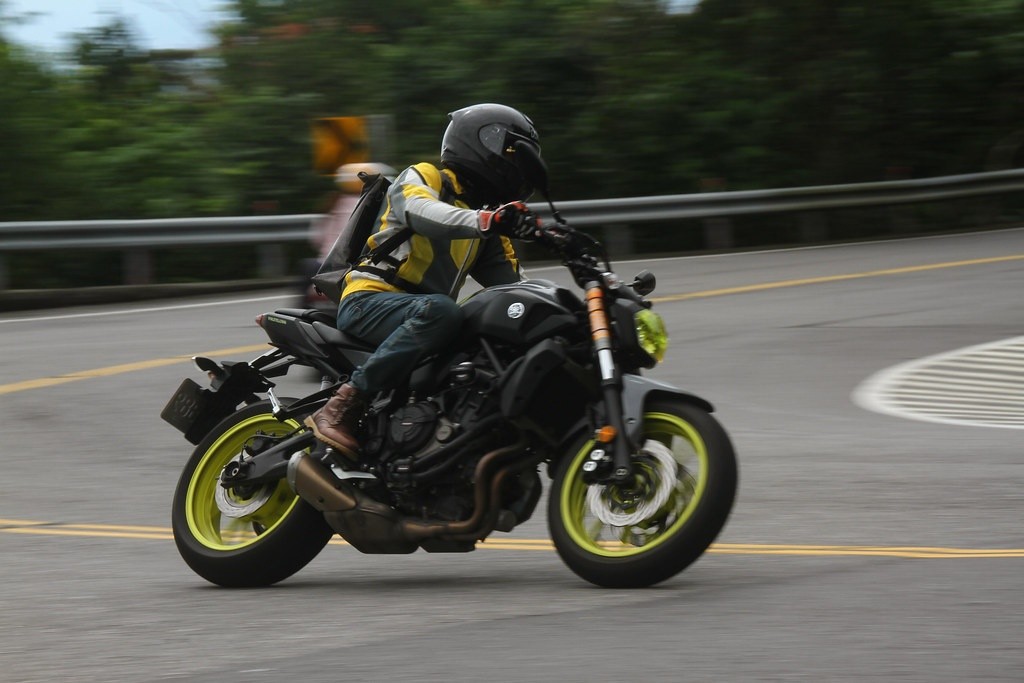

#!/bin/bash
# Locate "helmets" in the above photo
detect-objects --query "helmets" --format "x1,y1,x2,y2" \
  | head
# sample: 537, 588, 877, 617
442, 102, 541, 210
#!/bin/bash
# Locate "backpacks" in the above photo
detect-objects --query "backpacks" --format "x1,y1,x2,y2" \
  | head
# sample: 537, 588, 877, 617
311, 170, 454, 305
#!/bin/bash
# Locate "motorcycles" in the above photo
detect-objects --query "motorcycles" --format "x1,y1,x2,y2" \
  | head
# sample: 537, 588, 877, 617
157, 142, 740, 590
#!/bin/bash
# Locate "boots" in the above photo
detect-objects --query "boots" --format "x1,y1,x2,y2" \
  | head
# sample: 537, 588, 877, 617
304, 383, 370, 461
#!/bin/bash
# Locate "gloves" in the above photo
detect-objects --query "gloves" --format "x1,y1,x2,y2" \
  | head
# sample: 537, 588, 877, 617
478, 201, 544, 243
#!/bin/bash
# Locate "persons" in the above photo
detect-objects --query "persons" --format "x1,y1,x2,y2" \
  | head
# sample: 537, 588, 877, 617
304, 104, 549, 461
